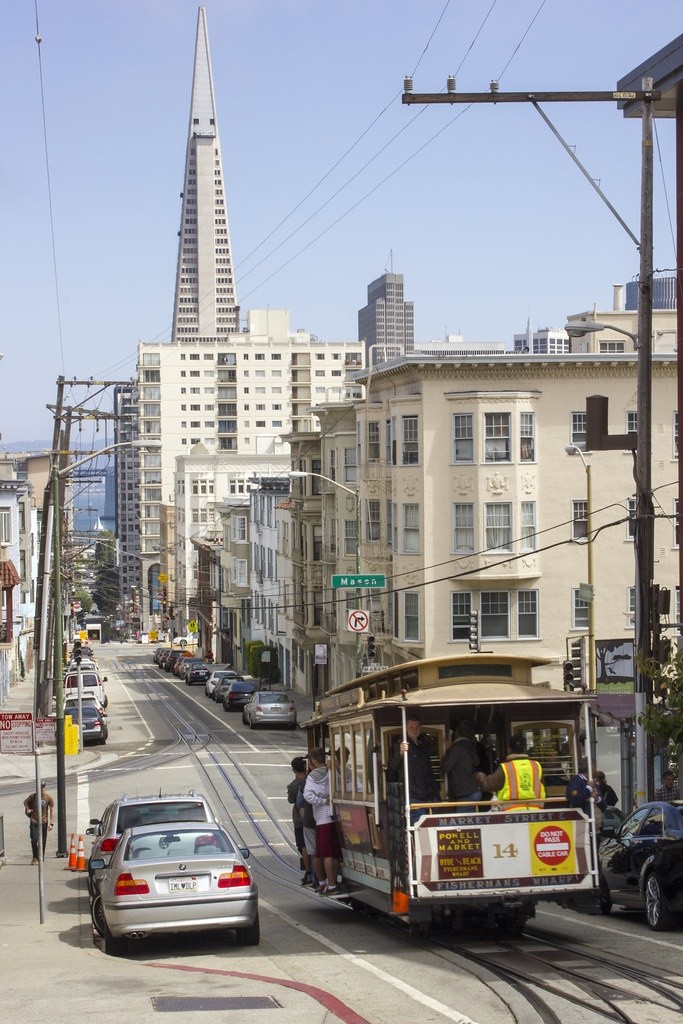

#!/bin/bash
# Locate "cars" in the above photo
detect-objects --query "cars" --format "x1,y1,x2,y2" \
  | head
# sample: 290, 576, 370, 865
90, 818, 262, 961
172, 632, 199, 646
242, 691, 298, 731
85, 789, 221, 895
64, 691, 108, 745
213, 675, 246, 703
598, 798, 683, 930
223, 682, 258, 712
602, 804, 628, 843
68, 645, 100, 672
205, 670, 238, 698
153, 647, 212, 686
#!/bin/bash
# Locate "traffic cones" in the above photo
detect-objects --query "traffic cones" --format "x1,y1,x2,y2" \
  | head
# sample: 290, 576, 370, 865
76, 835, 89, 871
64, 833, 77, 871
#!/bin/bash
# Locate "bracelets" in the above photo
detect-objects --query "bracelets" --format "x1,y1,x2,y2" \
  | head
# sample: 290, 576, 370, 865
50, 824, 54, 826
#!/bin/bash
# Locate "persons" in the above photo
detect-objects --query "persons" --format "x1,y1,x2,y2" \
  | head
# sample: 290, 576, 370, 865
287, 714, 619, 896
205, 651, 214, 664
654, 771, 680, 806
23, 781, 54, 865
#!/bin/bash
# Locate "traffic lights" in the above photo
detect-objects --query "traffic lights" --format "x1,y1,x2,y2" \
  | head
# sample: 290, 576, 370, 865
468, 610, 482, 651
562, 659, 575, 685
367, 634, 376, 659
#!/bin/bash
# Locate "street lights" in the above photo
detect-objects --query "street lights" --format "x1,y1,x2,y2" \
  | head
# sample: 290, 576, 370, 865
288, 469, 365, 678
51, 439, 164, 858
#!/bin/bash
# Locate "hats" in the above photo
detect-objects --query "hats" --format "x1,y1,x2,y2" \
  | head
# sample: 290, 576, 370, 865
308, 746, 325, 758
41, 782, 46, 789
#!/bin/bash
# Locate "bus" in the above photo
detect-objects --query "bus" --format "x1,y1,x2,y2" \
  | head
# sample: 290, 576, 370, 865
296, 654, 606, 945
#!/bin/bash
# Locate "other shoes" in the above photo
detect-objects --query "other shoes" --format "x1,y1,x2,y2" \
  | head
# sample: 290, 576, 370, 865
319, 886, 340, 897
299, 880, 312, 888
308, 882, 320, 889
30, 857, 37, 865
314, 885, 324, 893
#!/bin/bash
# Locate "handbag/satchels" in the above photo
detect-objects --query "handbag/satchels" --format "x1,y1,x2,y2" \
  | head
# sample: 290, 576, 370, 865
25, 794, 36, 818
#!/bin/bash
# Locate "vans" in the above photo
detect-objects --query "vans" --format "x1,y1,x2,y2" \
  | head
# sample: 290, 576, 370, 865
62, 673, 110, 710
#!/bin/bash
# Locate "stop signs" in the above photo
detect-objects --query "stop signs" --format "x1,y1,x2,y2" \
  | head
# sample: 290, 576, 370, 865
534, 825, 572, 866
